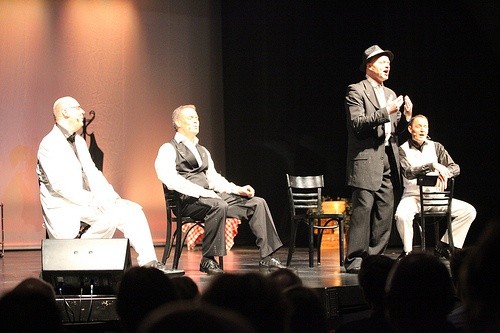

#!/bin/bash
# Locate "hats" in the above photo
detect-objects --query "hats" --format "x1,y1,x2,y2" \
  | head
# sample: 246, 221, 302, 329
360, 45, 393, 66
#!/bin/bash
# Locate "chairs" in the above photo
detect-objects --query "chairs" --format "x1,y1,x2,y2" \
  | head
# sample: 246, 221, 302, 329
412, 174, 456, 254
162, 182, 224, 271
286, 173, 347, 268
39, 178, 91, 240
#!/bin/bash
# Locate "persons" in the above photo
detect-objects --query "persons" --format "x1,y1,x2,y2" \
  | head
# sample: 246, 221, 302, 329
155, 104, 296, 275
0, 246, 500, 333
36, 96, 185, 275
395, 114, 477, 259
343, 45, 413, 274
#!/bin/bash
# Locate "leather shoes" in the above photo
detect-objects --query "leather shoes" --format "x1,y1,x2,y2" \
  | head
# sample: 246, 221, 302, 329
397, 250, 412, 261
260, 255, 286, 268
200, 258, 224, 274
434, 245, 453, 258
145, 260, 185, 275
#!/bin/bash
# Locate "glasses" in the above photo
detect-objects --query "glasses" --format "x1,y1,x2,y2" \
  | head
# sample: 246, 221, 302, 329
373, 61, 390, 64
71, 106, 81, 110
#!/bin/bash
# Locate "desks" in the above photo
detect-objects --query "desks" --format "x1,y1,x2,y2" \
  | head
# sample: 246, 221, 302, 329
181, 217, 242, 251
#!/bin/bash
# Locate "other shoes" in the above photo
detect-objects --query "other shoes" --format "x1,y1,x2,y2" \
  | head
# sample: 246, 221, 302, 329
346, 267, 360, 273
